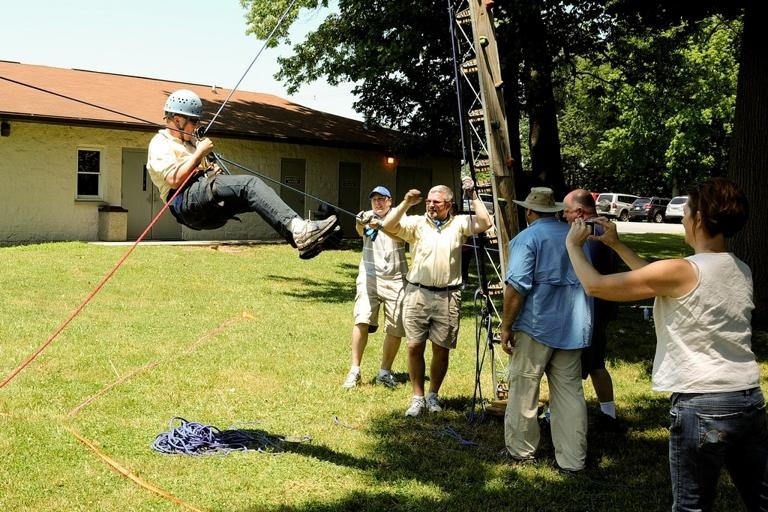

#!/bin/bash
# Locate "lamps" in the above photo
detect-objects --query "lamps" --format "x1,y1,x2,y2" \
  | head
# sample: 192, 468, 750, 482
387, 151, 394, 164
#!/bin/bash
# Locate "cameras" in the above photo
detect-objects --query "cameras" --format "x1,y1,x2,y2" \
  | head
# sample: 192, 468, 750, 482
584, 221, 596, 237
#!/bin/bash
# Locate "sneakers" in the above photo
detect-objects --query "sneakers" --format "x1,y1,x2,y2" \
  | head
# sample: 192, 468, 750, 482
299, 225, 341, 259
405, 397, 426, 417
497, 447, 536, 464
375, 374, 401, 389
588, 468, 636, 488
295, 214, 337, 248
342, 371, 361, 389
589, 414, 617, 437
551, 459, 584, 477
426, 393, 442, 413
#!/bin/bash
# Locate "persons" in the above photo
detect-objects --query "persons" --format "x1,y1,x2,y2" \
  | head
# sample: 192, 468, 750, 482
344, 185, 409, 392
538, 190, 616, 428
500, 187, 594, 477
565, 182, 768, 512
146, 89, 341, 259
382, 176, 492, 418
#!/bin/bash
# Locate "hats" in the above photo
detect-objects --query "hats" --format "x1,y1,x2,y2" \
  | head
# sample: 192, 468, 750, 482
368, 185, 391, 198
512, 186, 567, 213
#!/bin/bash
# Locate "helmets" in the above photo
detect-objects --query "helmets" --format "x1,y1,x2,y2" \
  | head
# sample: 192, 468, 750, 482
164, 89, 203, 118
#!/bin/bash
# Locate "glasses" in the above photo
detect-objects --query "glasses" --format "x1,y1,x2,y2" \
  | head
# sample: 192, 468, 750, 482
424, 199, 456, 204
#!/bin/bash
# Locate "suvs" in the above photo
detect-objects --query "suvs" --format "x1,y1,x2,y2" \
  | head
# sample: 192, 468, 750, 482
463, 193, 493, 213
595, 192, 640, 222
664, 196, 690, 219
630, 197, 671, 222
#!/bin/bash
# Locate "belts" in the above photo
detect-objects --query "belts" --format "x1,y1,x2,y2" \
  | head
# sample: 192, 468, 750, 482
413, 281, 463, 292
